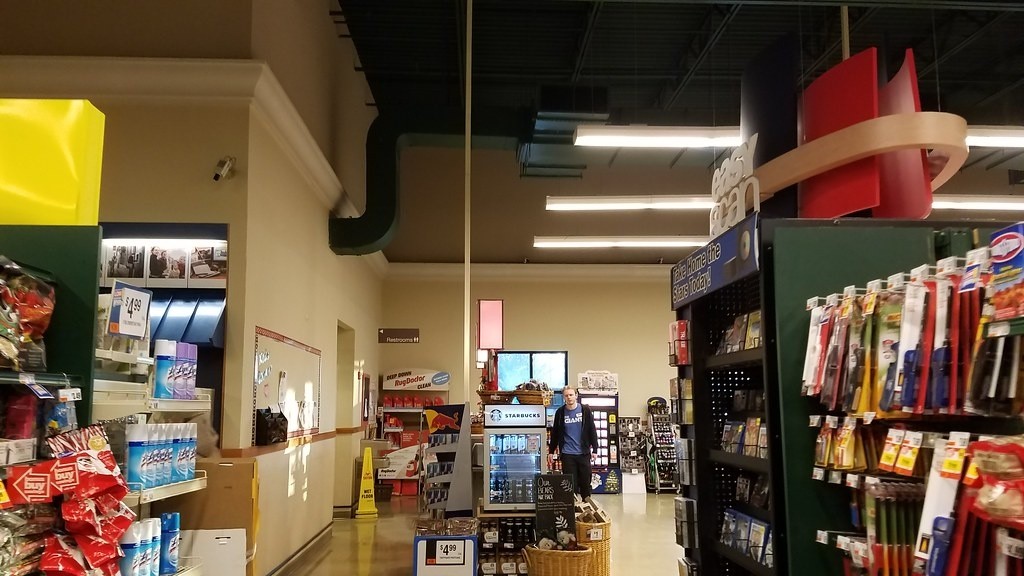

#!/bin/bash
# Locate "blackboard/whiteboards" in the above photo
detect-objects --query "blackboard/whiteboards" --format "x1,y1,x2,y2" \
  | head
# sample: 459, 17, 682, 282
534, 473, 575, 541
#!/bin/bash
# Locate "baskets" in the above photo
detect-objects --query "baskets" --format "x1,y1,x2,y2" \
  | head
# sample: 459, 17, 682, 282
478, 391, 514, 405
515, 390, 553, 405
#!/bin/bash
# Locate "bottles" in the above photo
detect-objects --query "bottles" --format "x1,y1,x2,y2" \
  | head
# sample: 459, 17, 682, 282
382, 392, 444, 409
481, 517, 533, 542
652, 403, 677, 480
478, 552, 528, 574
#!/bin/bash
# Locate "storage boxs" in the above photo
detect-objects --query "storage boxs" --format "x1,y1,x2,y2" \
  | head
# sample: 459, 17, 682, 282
360, 439, 392, 459
383, 393, 444, 496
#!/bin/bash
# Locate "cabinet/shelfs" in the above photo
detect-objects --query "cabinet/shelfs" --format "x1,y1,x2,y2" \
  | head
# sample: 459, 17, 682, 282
381, 368, 449, 450
425, 402, 473, 519
95, 348, 212, 576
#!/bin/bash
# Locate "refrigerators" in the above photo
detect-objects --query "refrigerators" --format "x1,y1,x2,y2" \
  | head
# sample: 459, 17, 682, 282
483, 403, 548, 511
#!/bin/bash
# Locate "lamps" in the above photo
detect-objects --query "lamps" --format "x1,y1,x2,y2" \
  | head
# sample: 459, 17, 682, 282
532, 234, 709, 248
544, 54, 1024, 211
571, 0, 1024, 149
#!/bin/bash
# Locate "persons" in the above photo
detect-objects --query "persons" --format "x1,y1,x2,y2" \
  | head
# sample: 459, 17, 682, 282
150, 246, 185, 279
125, 255, 135, 277
548, 385, 599, 503
759, 435, 767, 459
